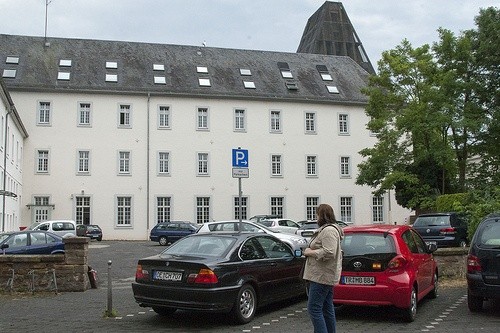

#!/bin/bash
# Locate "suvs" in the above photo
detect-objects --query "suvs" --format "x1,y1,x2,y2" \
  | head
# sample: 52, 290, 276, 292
296, 220, 347, 240
411, 213, 465, 248
149, 220, 198, 245
466, 213, 500, 313
260, 219, 300, 235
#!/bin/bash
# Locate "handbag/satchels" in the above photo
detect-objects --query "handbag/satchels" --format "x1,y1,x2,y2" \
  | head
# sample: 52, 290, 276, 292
299, 262, 310, 285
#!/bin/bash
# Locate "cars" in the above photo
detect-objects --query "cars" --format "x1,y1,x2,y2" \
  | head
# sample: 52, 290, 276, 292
131, 233, 306, 324
76, 225, 101, 241
0, 231, 64, 255
325, 223, 438, 323
196, 220, 310, 253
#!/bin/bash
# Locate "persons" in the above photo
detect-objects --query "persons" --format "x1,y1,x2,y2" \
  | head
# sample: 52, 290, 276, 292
301, 204, 343, 333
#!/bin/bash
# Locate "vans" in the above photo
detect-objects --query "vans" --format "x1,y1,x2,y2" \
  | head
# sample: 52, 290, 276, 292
249, 214, 287, 226
21, 220, 76, 240
